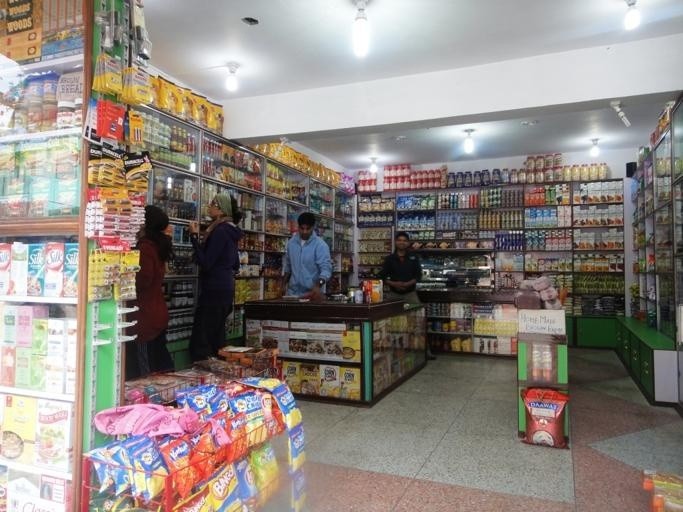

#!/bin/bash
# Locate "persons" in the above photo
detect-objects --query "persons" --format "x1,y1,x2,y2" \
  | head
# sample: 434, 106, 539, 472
185, 188, 243, 364
274, 212, 334, 304
125, 207, 175, 379
379, 232, 436, 363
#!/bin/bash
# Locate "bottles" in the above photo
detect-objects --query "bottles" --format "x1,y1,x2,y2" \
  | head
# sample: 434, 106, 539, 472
140, 109, 355, 343
382, 153, 607, 189
356, 170, 376, 192
531, 343, 542, 383
542, 345, 553, 383
437, 183, 524, 250
9, 73, 83, 130
654, 155, 682, 305
424, 302, 471, 354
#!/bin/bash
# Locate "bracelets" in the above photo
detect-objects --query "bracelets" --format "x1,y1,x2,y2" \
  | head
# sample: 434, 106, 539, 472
317, 277, 325, 288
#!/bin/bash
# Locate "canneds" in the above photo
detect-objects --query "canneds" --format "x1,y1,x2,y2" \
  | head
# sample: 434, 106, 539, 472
162, 281, 194, 343
347, 287, 355, 304
